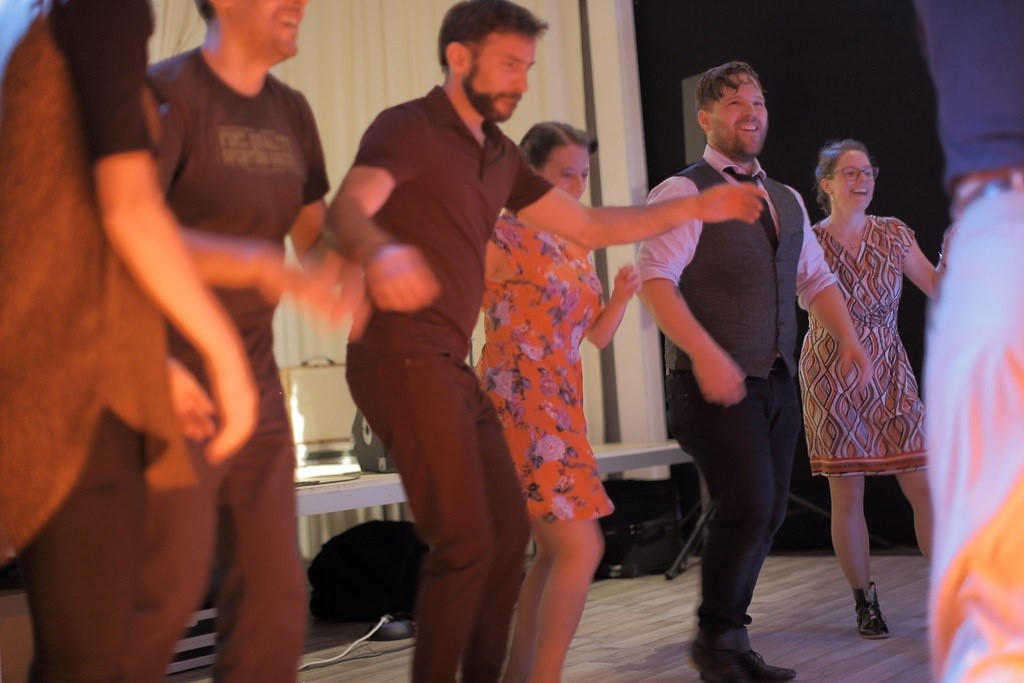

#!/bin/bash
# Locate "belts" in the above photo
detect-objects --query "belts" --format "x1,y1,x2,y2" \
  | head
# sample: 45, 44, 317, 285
952, 168, 1021, 218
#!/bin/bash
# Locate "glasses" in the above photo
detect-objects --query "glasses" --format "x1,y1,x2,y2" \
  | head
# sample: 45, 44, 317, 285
825, 166, 879, 181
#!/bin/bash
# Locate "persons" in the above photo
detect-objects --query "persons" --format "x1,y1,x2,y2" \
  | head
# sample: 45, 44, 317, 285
0, 0, 258, 683
473, 121, 641, 681
327, 0, 768, 683
127, 0, 370, 683
798, 140, 960, 638
634, 61, 873, 683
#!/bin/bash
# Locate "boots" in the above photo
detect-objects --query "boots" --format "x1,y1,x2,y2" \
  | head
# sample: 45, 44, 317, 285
853, 582, 891, 639
690, 626, 797, 683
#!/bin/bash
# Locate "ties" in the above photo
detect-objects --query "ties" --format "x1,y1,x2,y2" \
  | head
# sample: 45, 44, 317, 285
723, 166, 779, 258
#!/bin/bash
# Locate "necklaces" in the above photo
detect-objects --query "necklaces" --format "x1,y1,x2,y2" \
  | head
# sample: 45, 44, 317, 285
827, 217, 866, 249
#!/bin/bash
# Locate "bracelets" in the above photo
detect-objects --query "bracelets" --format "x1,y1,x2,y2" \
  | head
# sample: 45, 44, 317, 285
941, 258, 948, 270
